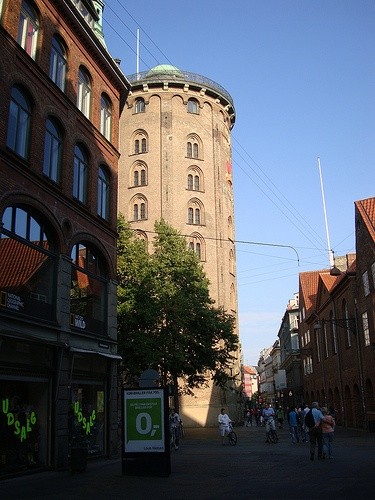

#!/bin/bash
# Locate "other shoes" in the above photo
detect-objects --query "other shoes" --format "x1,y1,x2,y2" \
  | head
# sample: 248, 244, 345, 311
323, 452, 326, 459
329, 457, 334, 459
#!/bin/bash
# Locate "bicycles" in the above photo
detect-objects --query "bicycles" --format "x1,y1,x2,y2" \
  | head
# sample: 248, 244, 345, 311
263, 420, 279, 444
289, 426, 298, 445
170, 425, 176, 452
220, 422, 239, 446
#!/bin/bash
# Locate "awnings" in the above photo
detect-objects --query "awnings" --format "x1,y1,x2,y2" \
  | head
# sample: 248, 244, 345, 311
71, 349, 121, 360
242, 365, 256, 375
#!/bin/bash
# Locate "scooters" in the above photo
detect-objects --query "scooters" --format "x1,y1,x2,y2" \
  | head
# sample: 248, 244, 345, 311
68, 433, 87, 472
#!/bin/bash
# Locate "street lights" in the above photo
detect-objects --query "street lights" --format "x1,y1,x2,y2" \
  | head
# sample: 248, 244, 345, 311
314, 318, 367, 432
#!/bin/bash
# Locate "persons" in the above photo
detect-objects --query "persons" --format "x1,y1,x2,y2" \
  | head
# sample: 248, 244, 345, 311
244, 400, 335, 460
218, 408, 232, 447
169, 410, 181, 449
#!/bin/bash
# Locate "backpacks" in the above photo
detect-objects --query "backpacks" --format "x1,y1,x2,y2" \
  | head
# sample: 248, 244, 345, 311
306, 409, 315, 428
